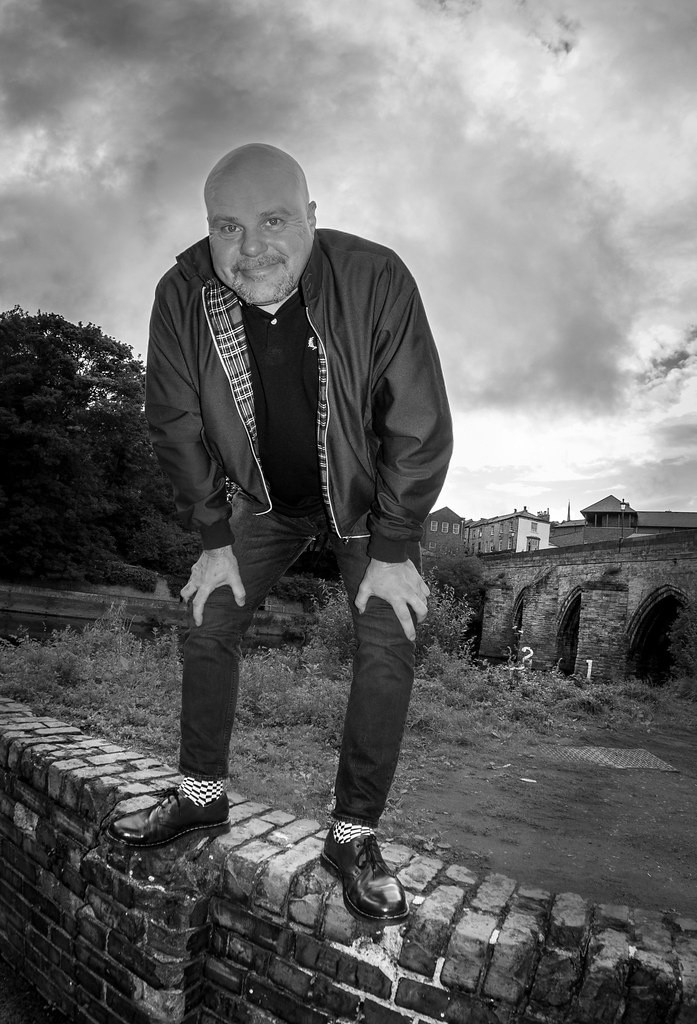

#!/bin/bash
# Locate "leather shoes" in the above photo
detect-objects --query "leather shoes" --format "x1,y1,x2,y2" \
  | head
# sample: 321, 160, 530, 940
105, 786, 231, 852
320, 821, 409, 925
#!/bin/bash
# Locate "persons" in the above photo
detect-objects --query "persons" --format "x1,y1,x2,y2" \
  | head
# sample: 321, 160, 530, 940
107, 144, 453, 927
